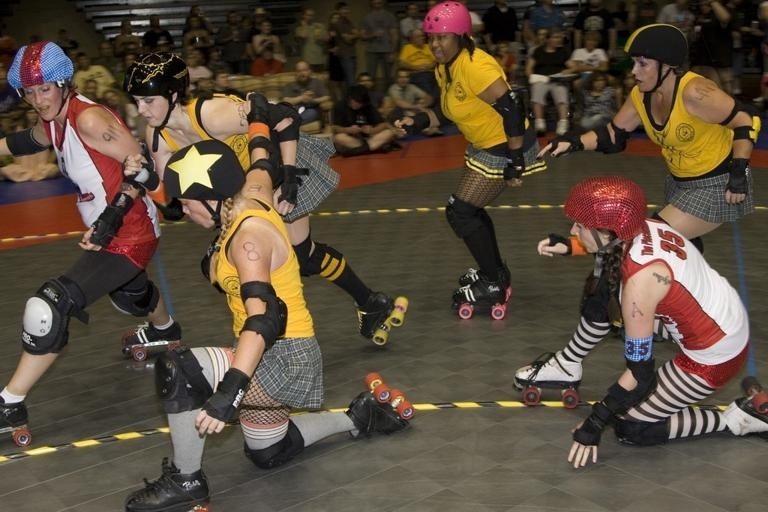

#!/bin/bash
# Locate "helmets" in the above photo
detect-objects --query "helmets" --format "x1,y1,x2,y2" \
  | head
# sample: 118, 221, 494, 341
7, 40, 74, 89
423, 1, 472, 36
123, 51, 190, 96
624, 23, 689, 65
163, 139, 245, 200
564, 175, 648, 240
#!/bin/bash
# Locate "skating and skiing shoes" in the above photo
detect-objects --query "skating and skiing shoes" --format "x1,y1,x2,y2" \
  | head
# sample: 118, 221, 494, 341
724, 376, 768, 436
514, 350, 583, 408
122, 321, 181, 362
124, 458, 209, 512
354, 292, 408, 345
0, 395, 33, 448
344, 372, 415, 439
452, 266, 512, 320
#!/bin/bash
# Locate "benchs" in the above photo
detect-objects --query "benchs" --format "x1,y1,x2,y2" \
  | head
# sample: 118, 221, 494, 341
76, 0, 306, 75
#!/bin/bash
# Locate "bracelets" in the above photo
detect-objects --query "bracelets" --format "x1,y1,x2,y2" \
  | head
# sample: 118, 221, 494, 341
502, 145, 526, 179
723, 156, 753, 194
124, 163, 160, 192
548, 131, 586, 157
205, 364, 254, 423
277, 161, 311, 205
399, 112, 433, 138
86, 190, 135, 249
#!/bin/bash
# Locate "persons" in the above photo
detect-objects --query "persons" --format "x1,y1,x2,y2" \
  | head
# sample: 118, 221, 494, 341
1, 0, 453, 176
116, 47, 397, 341
510, 173, 768, 470
123, 88, 412, 512
393, 0, 543, 306
462, 1, 768, 142
0, 40, 184, 433
534, 20, 764, 342
